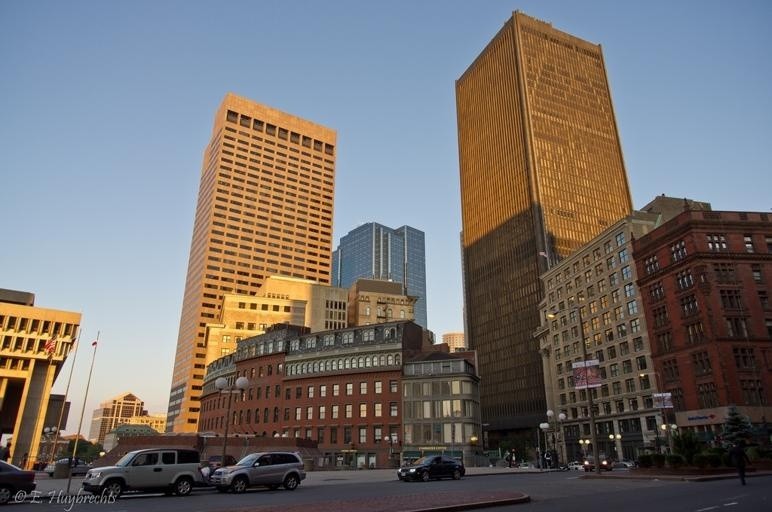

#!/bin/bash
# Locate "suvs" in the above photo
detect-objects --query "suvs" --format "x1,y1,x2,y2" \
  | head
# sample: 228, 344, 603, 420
81, 447, 202, 499
203, 452, 306, 492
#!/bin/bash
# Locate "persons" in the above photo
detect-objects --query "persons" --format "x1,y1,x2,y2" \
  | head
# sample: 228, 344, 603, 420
0, 442, 56, 477
506, 445, 552, 472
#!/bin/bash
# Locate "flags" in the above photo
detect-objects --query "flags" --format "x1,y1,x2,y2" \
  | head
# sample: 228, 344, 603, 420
44, 334, 57, 364
69, 336, 79, 353
92, 340, 97, 350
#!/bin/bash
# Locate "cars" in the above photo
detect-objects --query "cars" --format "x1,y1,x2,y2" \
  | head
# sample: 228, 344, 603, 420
567, 450, 635, 472
45, 458, 91, 476
0, 460, 35, 504
398, 455, 465, 481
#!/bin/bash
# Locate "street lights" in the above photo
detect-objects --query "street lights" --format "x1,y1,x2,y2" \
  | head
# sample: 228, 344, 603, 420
44, 426, 57, 455
546, 306, 600, 473
215, 377, 248, 467
540, 410, 566, 469
640, 372, 674, 456
